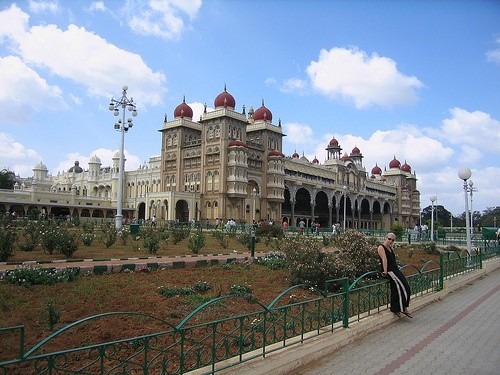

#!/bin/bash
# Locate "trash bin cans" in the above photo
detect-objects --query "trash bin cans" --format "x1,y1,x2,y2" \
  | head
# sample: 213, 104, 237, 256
427, 228, 447, 238
130, 224, 139, 234
482, 227, 498, 239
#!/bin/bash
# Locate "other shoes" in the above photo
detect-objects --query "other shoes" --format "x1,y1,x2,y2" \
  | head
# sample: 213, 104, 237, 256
395, 311, 406, 317
404, 310, 413, 318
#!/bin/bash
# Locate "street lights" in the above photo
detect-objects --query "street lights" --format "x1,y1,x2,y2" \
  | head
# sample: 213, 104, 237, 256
458, 167, 471, 265
467, 180, 478, 239
108, 85, 137, 230
430, 195, 437, 241
343, 185, 347, 229
250, 188, 261, 224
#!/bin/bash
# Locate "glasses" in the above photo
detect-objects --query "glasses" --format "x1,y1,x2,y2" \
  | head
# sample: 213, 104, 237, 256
388, 237, 395, 242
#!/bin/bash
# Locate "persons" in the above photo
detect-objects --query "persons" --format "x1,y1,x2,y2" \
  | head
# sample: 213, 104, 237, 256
495, 225, 500, 246
215, 217, 237, 233
299, 219, 305, 235
332, 222, 340, 236
311, 220, 320, 236
283, 220, 288, 232
124, 216, 145, 227
253, 218, 275, 232
150, 216, 157, 231
206, 218, 210, 230
377, 233, 414, 319
191, 217, 196, 230
420, 222, 428, 242
414, 224, 422, 242
